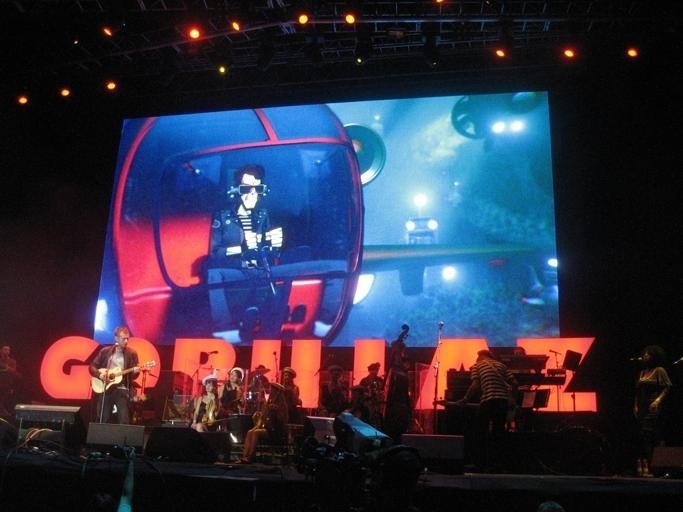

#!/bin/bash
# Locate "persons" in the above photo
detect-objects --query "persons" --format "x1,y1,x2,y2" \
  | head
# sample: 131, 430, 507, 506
208, 163, 308, 344
630, 345, 671, 479
0, 339, 21, 417
455, 348, 519, 473
189, 325, 414, 464
89, 325, 150, 426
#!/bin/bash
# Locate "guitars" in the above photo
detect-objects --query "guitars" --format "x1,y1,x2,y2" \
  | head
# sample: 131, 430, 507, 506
92, 360, 156, 394
436, 396, 516, 423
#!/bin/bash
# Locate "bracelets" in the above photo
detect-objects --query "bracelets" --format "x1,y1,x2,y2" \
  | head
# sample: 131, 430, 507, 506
656, 397, 662, 405
462, 395, 471, 400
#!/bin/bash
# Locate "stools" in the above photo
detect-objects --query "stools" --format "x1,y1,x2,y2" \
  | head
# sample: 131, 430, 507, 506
259, 423, 304, 465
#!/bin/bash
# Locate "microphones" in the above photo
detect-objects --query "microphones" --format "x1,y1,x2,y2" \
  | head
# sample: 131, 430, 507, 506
108, 343, 119, 354
208, 351, 218, 354
672, 357, 683, 365
438, 321, 444, 329
630, 357, 644, 361
549, 349, 561, 354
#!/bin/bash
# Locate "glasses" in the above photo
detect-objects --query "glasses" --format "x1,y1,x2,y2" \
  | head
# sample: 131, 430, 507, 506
237, 183, 264, 195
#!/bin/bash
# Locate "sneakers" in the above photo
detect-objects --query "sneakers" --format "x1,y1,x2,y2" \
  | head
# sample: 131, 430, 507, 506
234, 456, 252, 464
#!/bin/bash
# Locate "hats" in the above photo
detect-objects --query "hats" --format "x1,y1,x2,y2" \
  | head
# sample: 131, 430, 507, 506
255, 364, 270, 374
282, 366, 296, 378
229, 367, 244, 380
326, 362, 381, 397
201, 374, 218, 385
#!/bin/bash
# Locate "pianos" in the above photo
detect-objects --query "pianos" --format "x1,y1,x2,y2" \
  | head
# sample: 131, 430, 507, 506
14, 404, 81, 424
